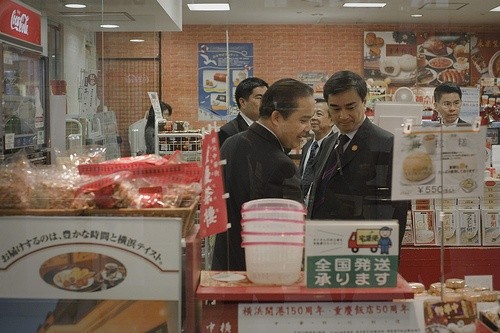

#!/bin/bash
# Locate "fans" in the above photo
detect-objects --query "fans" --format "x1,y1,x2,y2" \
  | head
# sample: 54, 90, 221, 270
392, 86, 416, 103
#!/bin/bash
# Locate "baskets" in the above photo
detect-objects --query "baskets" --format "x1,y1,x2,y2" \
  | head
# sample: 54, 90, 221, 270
68, 193, 201, 237
0, 206, 87, 215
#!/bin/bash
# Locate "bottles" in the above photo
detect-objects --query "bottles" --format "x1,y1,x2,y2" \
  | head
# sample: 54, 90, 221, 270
491, 121, 500, 175
160, 137, 201, 151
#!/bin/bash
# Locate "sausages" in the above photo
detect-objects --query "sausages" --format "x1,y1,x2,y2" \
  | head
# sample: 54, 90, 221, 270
441, 70, 465, 84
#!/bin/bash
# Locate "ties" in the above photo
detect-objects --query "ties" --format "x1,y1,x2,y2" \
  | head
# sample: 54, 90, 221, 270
302, 142, 318, 190
311, 134, 351, 219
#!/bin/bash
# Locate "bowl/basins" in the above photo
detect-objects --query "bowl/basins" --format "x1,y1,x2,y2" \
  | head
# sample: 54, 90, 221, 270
488, 50, 500, 78
240, 198, 309, 287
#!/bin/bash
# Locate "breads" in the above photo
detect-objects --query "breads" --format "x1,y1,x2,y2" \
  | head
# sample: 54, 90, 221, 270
403, 150, 432, 181
366, 33, 383, 57
408, 278, 496, 303
379, 54, 417, 76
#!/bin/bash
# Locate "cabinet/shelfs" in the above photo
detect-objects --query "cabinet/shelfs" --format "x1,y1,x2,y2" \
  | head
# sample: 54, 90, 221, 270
65, 114, 121, 157
153, 121, 204, 160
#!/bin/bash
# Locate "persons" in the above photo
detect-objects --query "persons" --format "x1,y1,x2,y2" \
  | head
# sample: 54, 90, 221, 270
433, 83, 467, 124
145, 102, 171, 151
309, 71, 407, 247
211, 78, 315, 270
297, 99, 336, 194
219, 76, 269, 144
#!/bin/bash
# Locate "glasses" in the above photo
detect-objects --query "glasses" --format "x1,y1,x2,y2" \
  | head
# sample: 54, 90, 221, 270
438, 100, 462, 110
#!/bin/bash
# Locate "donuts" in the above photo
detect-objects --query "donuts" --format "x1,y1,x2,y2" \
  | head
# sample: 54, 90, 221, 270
427, 41, 447, 55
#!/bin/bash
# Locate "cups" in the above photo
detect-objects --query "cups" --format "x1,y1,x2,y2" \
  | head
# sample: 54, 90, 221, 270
424, 134, 436, 154
176, 121, 184, 131
481, 95, 488, 105
4, 71, 18, 95
19, 84, 26, 97
488, 98, 495, 106
496, 98, 500, 106
166, 120, 172, 130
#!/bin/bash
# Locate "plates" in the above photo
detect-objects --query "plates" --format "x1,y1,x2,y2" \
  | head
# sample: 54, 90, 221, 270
437, 69, 470, 85
417, 58, 428, 67
428, 57, 453, 68
454, 62, 469, 70
416, 68, 437, 85
53, 270, 94, 289
400, 171, 436, 185
380, 56, 416, 84
417, 45, 453, 58
